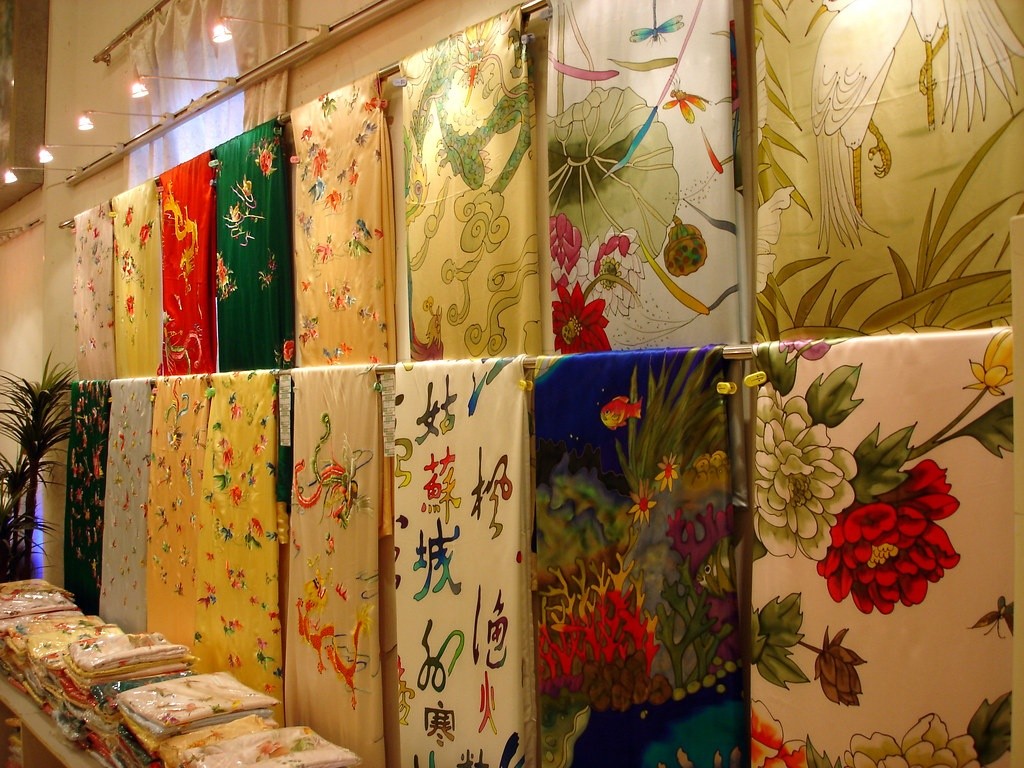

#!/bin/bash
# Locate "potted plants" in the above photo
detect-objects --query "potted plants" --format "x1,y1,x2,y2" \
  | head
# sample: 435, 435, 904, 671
0, 349, 79, 578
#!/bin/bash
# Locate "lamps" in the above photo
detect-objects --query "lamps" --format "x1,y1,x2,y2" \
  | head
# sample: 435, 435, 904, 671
76, 107, 174, 141
208, 11, 331, 50
36, 140, 126, 166
131, 72, 237, 100
4, 163, 85, 190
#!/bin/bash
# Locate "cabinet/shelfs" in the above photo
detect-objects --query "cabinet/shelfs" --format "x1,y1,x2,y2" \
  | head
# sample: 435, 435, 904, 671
0, 674, 104, 768
